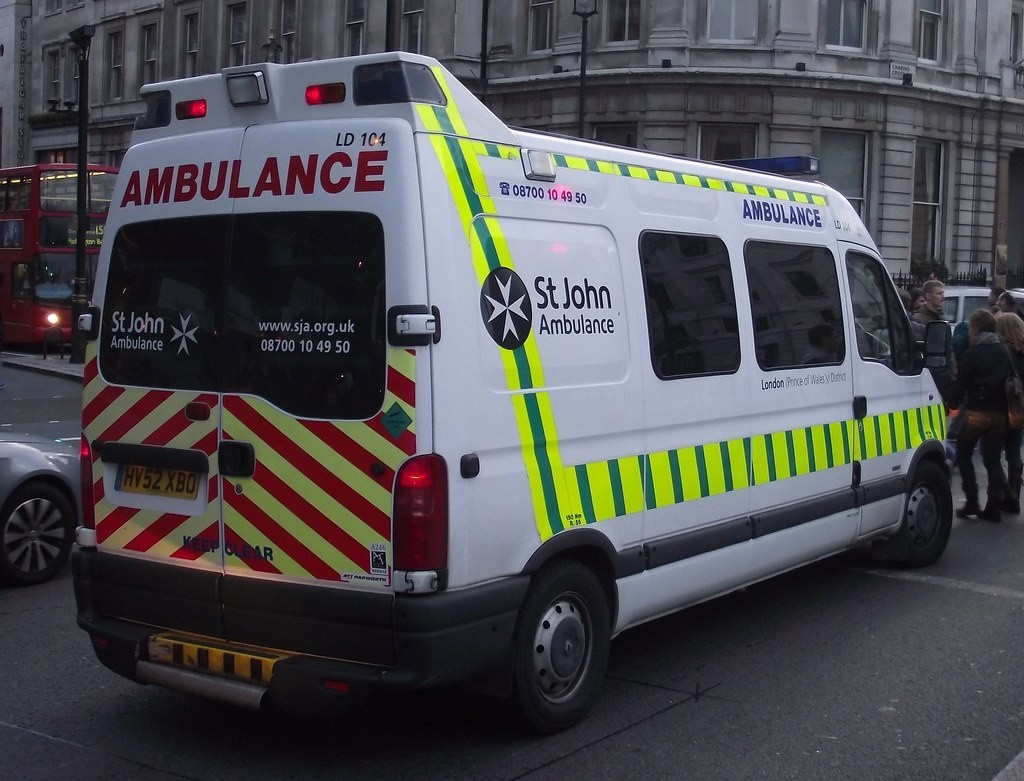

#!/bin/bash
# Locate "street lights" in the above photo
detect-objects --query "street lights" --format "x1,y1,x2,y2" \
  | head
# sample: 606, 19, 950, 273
65, 24, 97, 362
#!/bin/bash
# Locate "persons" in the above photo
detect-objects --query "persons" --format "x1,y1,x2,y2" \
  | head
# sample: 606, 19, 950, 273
802, 323, 839, 365
948, 287, 1024, 522
896, 281, 958, 423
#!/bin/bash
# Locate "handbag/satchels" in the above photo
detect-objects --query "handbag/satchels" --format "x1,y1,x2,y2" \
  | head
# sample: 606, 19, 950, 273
1006, 376, 1024, 430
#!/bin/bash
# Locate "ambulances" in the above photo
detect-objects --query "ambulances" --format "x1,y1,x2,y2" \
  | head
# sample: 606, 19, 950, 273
75, 52, 954, 732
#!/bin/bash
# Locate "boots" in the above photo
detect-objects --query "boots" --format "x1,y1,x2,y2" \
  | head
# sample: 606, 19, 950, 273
955, 483, 982, 517
977, 486, 1005, 522
1004, 485, 1021, 514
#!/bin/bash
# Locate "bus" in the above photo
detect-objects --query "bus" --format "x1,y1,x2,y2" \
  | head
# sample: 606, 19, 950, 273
0, 162, 120, 353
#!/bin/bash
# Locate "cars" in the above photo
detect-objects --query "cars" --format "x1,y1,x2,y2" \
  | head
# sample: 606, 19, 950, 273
0, 429, 80, 586
938, 284, 1024, 338
